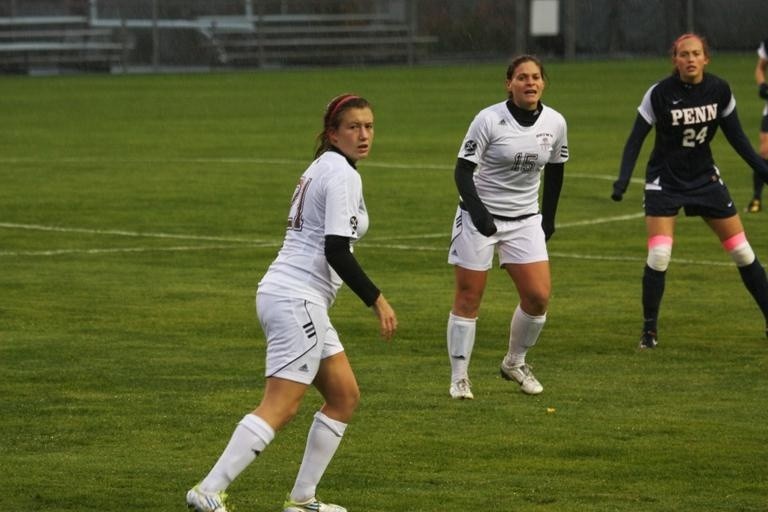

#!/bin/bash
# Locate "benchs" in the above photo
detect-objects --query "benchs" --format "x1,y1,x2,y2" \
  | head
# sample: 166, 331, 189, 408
187, 13, 439, 68
0, 15, 136, 74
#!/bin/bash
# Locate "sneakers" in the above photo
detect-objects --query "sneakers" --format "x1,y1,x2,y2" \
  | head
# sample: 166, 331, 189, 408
639, 330, 657, 350
185, 483, 230, 512
284, 491, 347, 512
450, 376, 474, 399
742, 198, 762, 214
500, 355, 544, 396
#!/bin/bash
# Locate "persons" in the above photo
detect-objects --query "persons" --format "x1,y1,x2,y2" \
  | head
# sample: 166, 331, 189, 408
749, 35, 768, 213
186, 96, 398, 511
610, 32, 768, 348
447, 56, 571, 401
